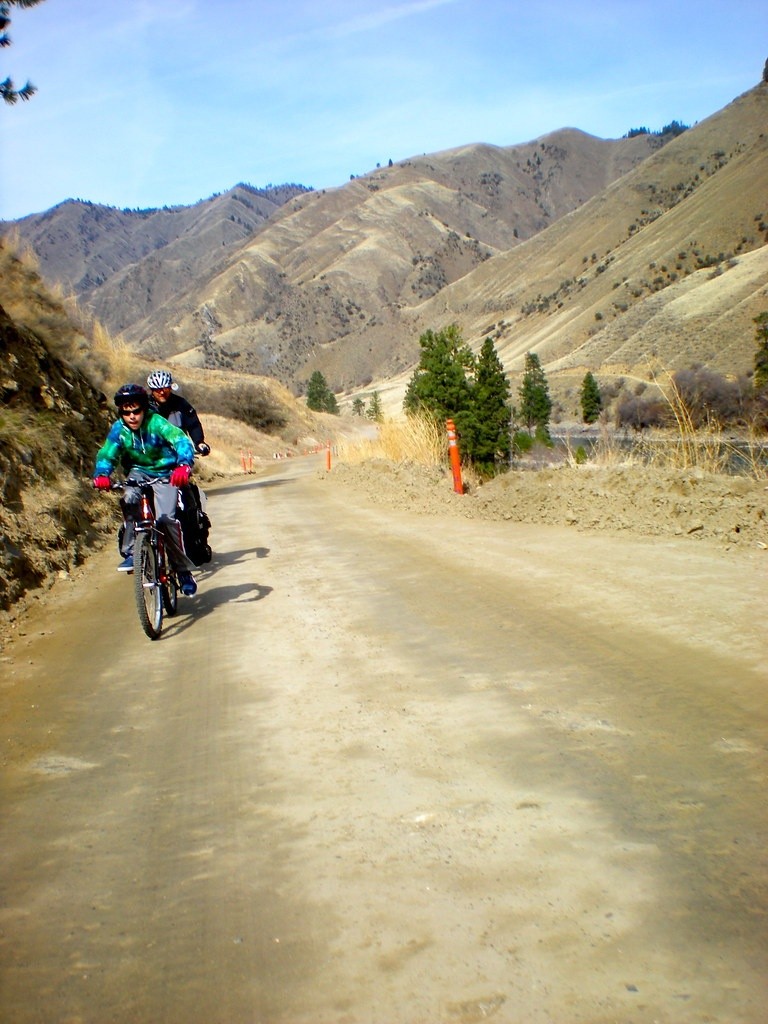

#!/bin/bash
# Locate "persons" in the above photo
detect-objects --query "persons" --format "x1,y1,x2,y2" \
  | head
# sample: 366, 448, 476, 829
146, 371, 212, 564
94, 383, 196, 596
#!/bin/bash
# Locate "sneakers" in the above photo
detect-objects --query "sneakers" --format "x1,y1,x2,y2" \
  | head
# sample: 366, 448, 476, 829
177, 570, 198, 598
117, 555, 133, 572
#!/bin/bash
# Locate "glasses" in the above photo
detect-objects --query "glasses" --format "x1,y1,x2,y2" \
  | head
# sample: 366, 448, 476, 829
119, 405, 144, 415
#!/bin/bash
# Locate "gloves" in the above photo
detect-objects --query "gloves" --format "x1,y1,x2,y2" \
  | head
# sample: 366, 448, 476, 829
196, 442, 210, 456
170, 464, 191, 487
93, 476, 110, 492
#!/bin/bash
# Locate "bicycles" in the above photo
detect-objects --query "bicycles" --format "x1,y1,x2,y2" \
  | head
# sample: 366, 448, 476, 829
92, 475, 187, 640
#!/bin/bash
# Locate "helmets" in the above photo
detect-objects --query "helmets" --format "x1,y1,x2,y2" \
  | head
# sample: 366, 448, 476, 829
114, 384, 147, 406
147, 371, 172, 389
